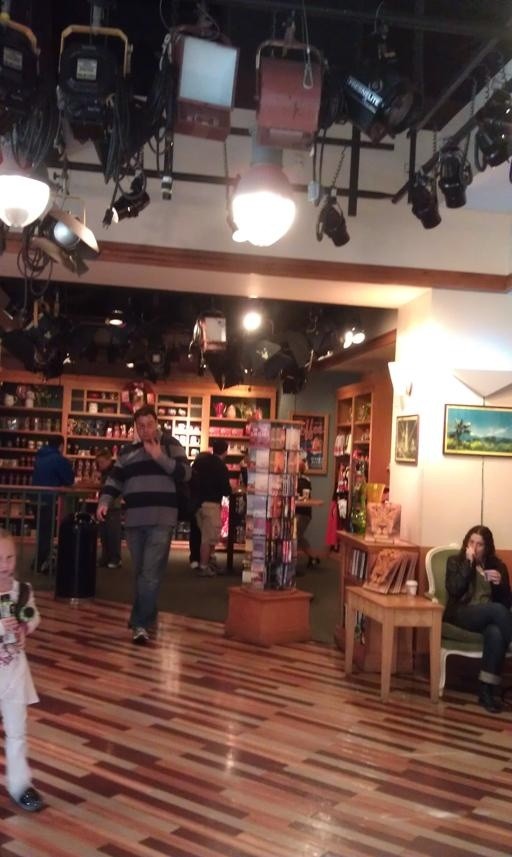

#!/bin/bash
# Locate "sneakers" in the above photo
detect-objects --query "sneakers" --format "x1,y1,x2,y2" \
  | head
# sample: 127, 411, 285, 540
108, 559, 122, 568
199, 564, 216, 577
9, 786, 44, 813
209, 561, 224, 574
41, 562, 56, 574
191, 566, 200, 575
307, 554, 321, 568
132, 625, 150, 642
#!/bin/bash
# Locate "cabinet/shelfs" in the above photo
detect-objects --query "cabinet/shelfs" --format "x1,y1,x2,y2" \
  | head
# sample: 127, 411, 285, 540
0, 370, 276, 545
334, 377, 390, 530
335, 528, 419, 670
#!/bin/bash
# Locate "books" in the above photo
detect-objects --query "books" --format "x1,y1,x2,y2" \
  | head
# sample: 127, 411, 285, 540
348, 547, 418, 595
340, 606, 368, 648
243, 422, 298, 599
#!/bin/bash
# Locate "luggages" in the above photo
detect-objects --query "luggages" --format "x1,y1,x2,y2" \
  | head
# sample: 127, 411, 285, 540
54, 510, 97, 598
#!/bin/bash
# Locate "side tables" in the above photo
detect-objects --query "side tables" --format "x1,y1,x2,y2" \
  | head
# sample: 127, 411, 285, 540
342, 585, 445, 703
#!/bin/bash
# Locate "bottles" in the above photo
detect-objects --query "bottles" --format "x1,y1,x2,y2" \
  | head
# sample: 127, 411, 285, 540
67, 418, 134, 484
18, 605, 34, 623
0, 437, 36, 485
0, 594, 17, 646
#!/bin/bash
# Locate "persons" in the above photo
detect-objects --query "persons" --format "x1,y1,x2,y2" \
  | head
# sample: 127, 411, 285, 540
294, 461, 321, 570
95, 405, 193, 645
179, 438, 233, 578
93, 451, 125, 568
0, 528, 46, 811
30, 435, 76, 573
442, 524, 511, 715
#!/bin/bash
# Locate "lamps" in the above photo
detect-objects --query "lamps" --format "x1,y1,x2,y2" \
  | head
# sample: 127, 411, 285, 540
406, 82, 510, 232
110, 173, 152, 223
229, 140, 351, 249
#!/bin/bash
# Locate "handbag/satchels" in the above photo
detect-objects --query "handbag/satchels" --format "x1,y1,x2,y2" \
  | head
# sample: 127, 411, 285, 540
179, 483, 199, 522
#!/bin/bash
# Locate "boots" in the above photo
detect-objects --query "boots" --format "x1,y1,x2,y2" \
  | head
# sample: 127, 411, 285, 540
478, 683, 501, 712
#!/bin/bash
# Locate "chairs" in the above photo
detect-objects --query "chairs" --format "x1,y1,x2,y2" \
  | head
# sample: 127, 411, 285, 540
423, 544, 510, 699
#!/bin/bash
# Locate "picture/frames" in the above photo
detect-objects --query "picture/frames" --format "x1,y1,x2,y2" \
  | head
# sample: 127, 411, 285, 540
395, 415, 419, 464
442, 404, 512, 457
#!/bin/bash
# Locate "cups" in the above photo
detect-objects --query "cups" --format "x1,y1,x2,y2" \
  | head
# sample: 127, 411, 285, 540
405, 580, 418, 598
